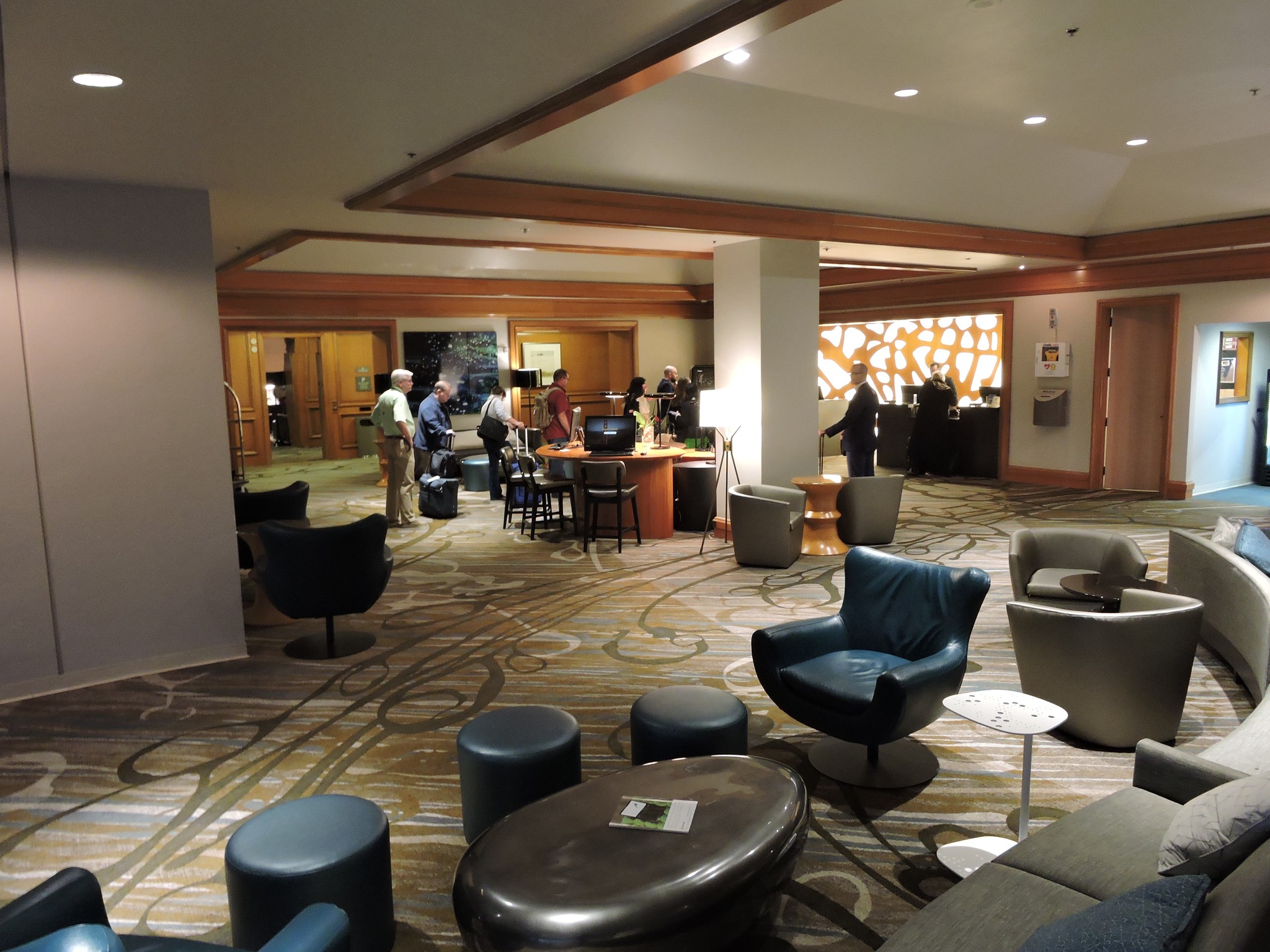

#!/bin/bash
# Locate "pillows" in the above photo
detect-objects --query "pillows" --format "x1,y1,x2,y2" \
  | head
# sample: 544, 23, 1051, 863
1233, 516, 1270, 578
1156, 768, 1270, 889
1015, 873, 1212, 952
1211, 515, 1244, 555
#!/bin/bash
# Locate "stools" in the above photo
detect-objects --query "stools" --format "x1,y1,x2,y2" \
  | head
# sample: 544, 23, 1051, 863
628, 682, 749, 771
218, 791, 399, 952
457, 703, 584, 847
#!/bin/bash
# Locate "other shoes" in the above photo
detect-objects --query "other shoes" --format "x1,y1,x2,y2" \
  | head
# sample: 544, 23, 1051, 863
388, 521, 402, 528
401, 521, 422, 528
552, 491, 570, 498
493, 495, 506, 501
907, 468, 918, 475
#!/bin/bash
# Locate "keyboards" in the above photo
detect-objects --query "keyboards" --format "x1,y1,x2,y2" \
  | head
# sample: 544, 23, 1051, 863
548, 443, 565, 449
589, 451, 634, 456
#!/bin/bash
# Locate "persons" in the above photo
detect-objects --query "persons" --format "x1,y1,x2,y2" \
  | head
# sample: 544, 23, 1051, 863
370, 369, 424, 529
818, 364, 880, 478
415, 365, 697, 474
905, 363, 958, 476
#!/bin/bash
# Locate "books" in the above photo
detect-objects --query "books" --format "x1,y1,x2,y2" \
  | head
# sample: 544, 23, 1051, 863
609, 795, 698, 833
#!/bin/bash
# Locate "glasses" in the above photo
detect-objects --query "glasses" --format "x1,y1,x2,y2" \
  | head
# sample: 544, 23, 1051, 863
560, 377, 570, 381
849, 372, 865, 376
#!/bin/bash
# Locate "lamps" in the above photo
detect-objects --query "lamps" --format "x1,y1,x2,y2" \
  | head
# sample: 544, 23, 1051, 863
642, 391, 677, 450
599, 390, 630, 416
516, 367, 544, 428
698, 387, 747, 554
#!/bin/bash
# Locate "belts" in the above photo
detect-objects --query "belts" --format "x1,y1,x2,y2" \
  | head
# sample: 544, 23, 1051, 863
385, 435, 411, 453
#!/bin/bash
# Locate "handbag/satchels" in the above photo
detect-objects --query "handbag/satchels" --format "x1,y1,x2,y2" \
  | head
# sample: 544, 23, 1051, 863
430, 449, 463, 479
840, 439, 846, 456
651, 415, 671, 434
477, 415, 509, 446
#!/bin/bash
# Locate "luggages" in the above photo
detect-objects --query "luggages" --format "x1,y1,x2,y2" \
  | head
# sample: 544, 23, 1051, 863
511, 425, 542, 507
418, 433, 459, 518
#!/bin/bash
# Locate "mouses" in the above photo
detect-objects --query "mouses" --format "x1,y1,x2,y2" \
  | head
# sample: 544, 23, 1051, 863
554, 447, 562, 450
640, 451, 647, 455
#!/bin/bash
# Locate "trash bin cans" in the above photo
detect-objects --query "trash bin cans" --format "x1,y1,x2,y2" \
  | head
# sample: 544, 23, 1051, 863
354, 416, 379, 458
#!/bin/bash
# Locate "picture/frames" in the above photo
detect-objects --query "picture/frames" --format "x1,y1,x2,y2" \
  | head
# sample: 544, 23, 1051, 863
521, 340, 563, 388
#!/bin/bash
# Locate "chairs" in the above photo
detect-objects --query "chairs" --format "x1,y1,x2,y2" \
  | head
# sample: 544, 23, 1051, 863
0, 866, 354, 952
751, 524, 1270, 952
232, 479, 395, 662
497, 445, 643, 554
836, 473, 907, 546
728, 483, 808, 570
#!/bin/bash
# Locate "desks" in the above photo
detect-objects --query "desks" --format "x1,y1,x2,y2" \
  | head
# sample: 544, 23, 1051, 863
790, 473, 851, 557
1058, 569, 1182, 617
671, 447, 718, 533
452, 752, 812, 952
236, 516, 312, 627
460, 452, 493, 493
933, 687, 1071, 882
535, 440, 688, 541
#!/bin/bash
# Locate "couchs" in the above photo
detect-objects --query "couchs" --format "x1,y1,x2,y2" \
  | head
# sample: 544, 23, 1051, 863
412, 413, 542, 457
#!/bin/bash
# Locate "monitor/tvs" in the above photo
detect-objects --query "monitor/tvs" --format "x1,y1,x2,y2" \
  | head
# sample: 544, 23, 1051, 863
564, 406, 584, 448
583, 415, 637, 451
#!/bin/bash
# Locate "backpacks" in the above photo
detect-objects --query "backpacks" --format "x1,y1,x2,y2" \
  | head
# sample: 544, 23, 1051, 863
534, 386, 564, 428
